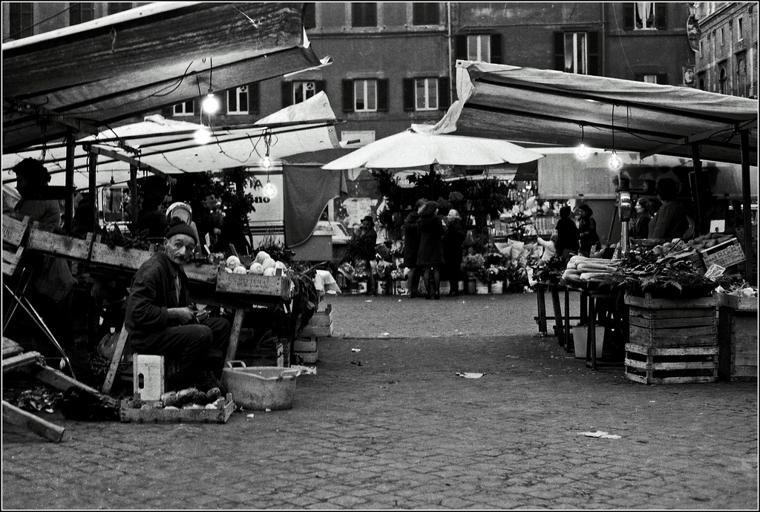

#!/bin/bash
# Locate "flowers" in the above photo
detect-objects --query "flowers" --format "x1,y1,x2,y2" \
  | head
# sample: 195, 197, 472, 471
461, 196, 543, 288
338, 240, 411, 283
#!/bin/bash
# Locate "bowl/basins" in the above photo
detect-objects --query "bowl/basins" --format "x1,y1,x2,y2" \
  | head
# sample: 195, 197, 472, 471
222, 357, 301, 411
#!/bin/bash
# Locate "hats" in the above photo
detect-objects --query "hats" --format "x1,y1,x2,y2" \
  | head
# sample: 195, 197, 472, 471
361, 216, 373, 223
166, 222, 197, 244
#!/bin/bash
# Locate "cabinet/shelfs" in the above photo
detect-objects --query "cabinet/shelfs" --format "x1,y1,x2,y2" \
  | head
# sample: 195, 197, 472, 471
530, 280, 629, 370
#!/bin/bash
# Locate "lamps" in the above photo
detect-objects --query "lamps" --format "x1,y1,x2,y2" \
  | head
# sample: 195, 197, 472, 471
609, 102, 624, 171
261, 133, 278, 198
575, 121, 589, 161
201, 56, 220, 117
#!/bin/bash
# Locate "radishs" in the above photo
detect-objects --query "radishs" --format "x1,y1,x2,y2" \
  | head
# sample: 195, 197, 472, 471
224, 251, 287, 276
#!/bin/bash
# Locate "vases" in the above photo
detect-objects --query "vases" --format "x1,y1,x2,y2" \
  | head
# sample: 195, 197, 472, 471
356, 280, 504, 296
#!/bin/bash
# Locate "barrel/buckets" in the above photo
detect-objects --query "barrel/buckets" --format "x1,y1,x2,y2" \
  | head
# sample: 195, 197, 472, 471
573, 324, 605, 359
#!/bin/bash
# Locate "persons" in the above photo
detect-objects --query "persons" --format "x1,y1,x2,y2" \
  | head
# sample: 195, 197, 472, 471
11, 157, 60, 230
123, 222, 232, 388
348, 215, 377, 270
648, 178, 687, 240
555, 204, 601, 260
633, 197, 652, 239
404, 189, 466, 300
119, 188, 131, 212
